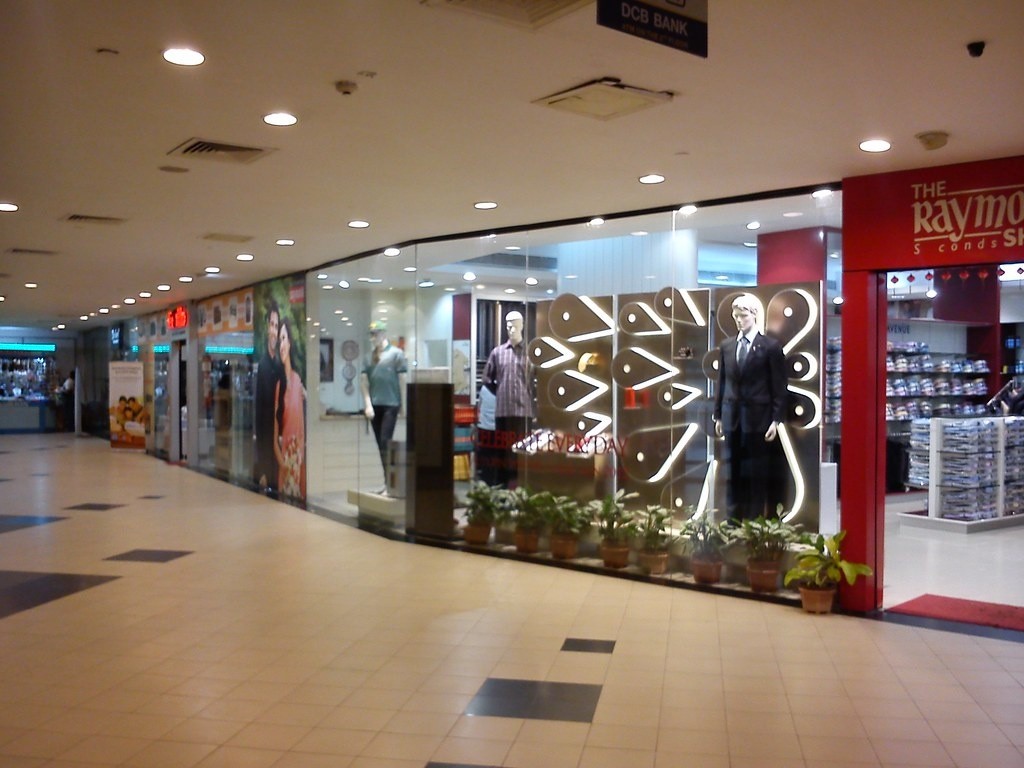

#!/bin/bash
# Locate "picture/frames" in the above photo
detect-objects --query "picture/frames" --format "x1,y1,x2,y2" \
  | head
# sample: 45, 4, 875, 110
320, 339, 334, 383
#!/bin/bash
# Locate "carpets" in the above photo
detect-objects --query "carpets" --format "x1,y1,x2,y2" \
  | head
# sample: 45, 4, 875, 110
884, 593, 1024, 631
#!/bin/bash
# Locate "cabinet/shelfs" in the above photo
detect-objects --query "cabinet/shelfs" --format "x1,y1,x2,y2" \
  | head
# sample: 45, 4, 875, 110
824, 349, 991, 425
895, 417, 1024, 535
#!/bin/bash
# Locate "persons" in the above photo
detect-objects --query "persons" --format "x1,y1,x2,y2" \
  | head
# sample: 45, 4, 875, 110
110, 395, 145, 433
62, 371, 77, 432
360, 320, 408, 497
710, 295, 786, 527
482, 311, 535, 489
256, 308, 281, 490
1009, 388, 1024, 416
477, 380, 496, 487
274, 316, 307, 502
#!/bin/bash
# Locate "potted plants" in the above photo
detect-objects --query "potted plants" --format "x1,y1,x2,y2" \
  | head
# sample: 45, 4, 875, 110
780, 530, 874, 613
630, 496, 684, 572
680, 503, 738, 583
589, 487, 641, 566
463, 479, 504, 544
725, 503, 806, 594
491, 490, 518, 543
543, 493, 598, 557
513, 487, 578, 552
